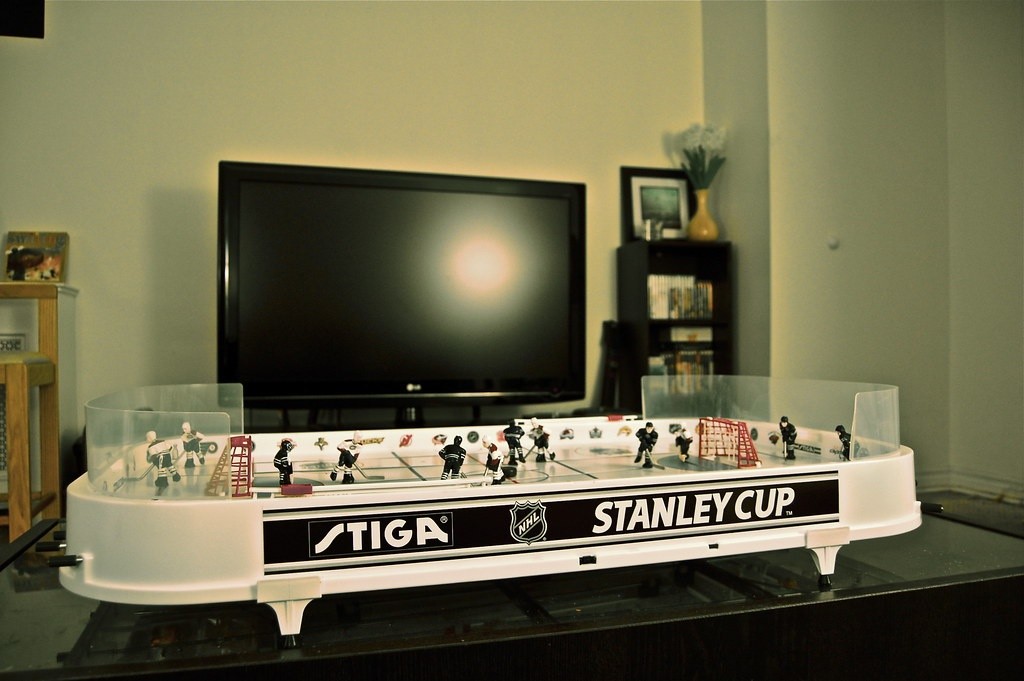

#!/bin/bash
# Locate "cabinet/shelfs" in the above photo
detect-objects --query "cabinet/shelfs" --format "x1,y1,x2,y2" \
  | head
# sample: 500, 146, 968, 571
617, 240, 736, 419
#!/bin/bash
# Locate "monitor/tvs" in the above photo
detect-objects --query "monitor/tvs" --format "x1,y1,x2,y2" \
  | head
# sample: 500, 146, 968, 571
215, 155, 588, 432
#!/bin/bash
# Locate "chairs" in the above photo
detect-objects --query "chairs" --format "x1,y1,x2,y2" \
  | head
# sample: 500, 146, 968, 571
0, 283, 57, 569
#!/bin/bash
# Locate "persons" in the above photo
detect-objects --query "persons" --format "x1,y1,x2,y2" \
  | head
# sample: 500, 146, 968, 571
273, 440, 293, 485
182, 422, 204, 467
531, 417, 548, 462
675, 428, 693, 462
780, 416, 797, 460
836, 425, 860, 461
482, 437, 505, 485
634, 422, 658, 467
439, 436, 466, 479
146, 431, 180, 486
330, 430, 363, 484
503, 419, 525, 465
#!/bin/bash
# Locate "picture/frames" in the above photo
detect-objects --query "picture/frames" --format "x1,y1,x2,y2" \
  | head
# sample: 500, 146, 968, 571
621, 164, 697, 244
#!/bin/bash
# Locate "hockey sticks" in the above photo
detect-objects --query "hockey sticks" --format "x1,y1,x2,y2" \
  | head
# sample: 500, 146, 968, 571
482, 467, 489, 476
784, 440, 789, 461
173, 450, 185, 465
504, 451, 510, 459
514, 444, 535, 460
126, 463, 154, 481
353, 462, 386, 480
461, 472, 481, 486
646, 448, 665, 470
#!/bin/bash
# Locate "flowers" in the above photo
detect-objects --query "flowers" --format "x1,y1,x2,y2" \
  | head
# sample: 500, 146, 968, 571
676, 121, 730, 190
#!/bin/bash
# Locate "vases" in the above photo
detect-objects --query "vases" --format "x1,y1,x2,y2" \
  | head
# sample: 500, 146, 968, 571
687, 188, 718, 240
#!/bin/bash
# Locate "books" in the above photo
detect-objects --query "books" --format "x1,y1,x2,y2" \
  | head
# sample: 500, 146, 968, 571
649, 351, 712, 394
671, 327, 713, 341
647, 275, 713, 319
